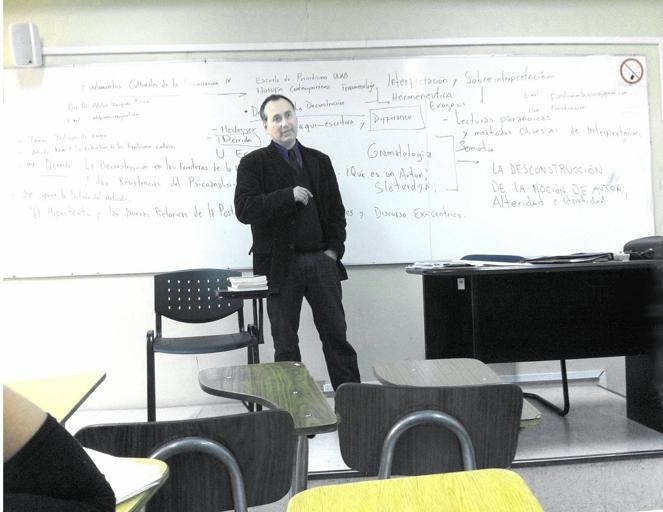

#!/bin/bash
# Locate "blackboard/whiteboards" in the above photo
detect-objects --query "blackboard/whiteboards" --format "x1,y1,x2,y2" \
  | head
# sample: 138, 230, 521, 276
0, 37, 657, 279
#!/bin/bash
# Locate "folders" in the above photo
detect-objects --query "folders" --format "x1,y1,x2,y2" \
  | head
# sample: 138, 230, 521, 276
526, 252, 614, 264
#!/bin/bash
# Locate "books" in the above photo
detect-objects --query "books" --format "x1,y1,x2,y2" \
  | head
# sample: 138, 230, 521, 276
226, 274, 269, 292
82, 446, 162, 505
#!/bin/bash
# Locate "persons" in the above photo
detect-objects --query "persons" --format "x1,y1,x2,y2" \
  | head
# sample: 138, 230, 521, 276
234, 95, 362, 392
3, 386, 117, 512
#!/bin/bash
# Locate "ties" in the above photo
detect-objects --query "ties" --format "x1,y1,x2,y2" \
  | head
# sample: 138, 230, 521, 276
288, 149, 300, 176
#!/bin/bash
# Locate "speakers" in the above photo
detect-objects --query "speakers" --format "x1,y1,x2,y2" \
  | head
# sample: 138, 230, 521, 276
11, 22, 41, 67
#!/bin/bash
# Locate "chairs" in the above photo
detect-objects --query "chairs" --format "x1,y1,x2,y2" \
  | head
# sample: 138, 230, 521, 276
145, 269, 267, 421
457, 254, 570, 416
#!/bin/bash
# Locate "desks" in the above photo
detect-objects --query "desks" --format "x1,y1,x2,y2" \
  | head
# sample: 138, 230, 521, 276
406, 259, 663, 436
